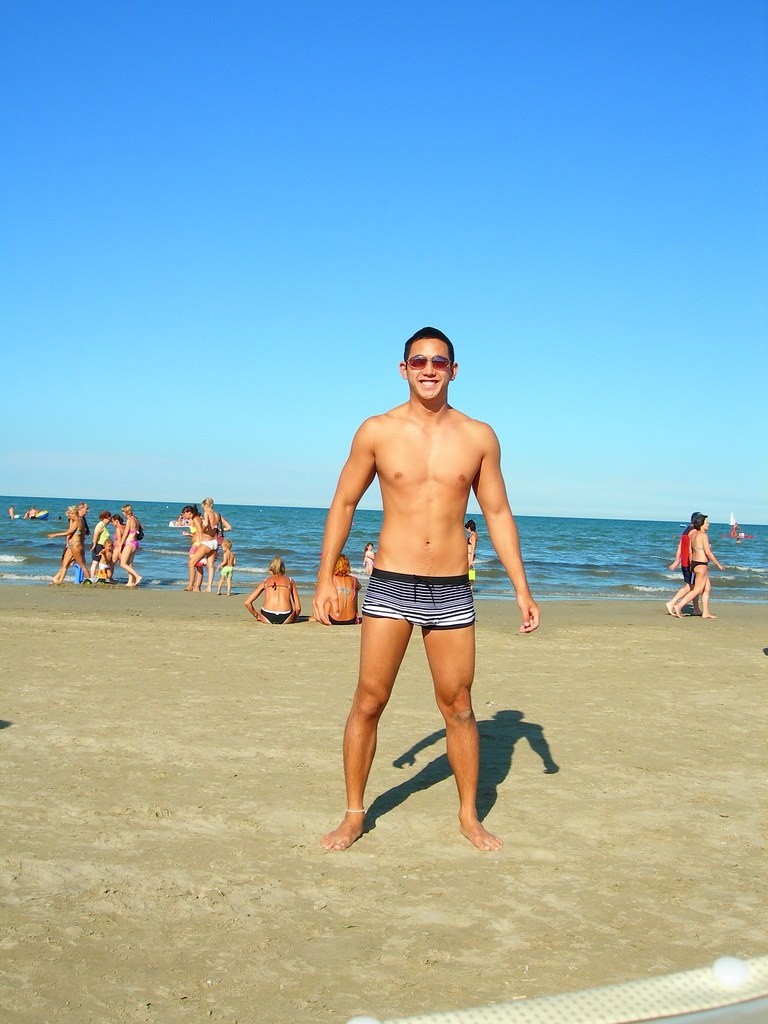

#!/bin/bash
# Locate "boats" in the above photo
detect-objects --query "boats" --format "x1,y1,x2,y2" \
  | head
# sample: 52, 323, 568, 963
34, 510, 49, 521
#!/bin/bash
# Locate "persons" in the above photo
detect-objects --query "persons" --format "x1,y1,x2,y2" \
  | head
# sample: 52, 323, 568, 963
47, 502, 144, 587
216, 540, 236, 595
182, 498, 231, 592
464, 520, 478, 593
363, 543, 375, 575
9, 506, 15, 520
737, 538, 741, 543
308, 554, 361, 625
731, 523, 745, 536
25, 506, 40, 519
311, 327, 540, 851
244, 556, 301, 624
665, 512, 725, 619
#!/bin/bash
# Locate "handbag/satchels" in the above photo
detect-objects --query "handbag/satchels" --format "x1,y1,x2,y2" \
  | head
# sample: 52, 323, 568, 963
218, 514, 224, 546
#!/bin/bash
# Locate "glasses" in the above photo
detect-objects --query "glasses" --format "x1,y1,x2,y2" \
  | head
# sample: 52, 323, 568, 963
406, 355, 453, 369
